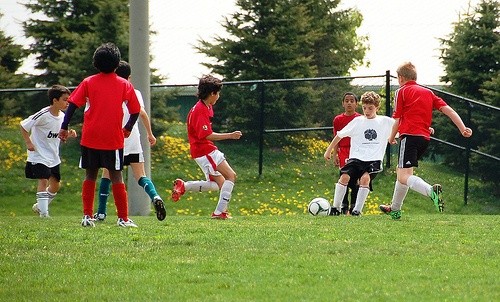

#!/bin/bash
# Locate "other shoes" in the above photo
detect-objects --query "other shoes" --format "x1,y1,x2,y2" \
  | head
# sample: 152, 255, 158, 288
93, 213, 106, 222
351, 210, 361, 216
32, 203, 50, 218
116, 218, 138, 228
430, 184, 444, 212
328, 207, 341, 216
127, 218, 133, 223
172, 179, 185, 202
82, 215, 96, 227
379, 205, 401, 219
341, 203, 355, 215
211, 211, 233, 219
152, 196, 167, 221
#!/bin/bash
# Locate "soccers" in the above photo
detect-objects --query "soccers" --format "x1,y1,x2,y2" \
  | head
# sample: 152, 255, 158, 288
308, 198, 331, 216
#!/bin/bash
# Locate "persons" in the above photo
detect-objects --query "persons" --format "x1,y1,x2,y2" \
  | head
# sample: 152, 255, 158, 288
378, 63, 473, 220
19, 85, 77, 216
172, 76, 242, 220
59, 43, 140, 228
94, 62, 166, 222
323, 91, 434, 217
333, 92, 359, 214
